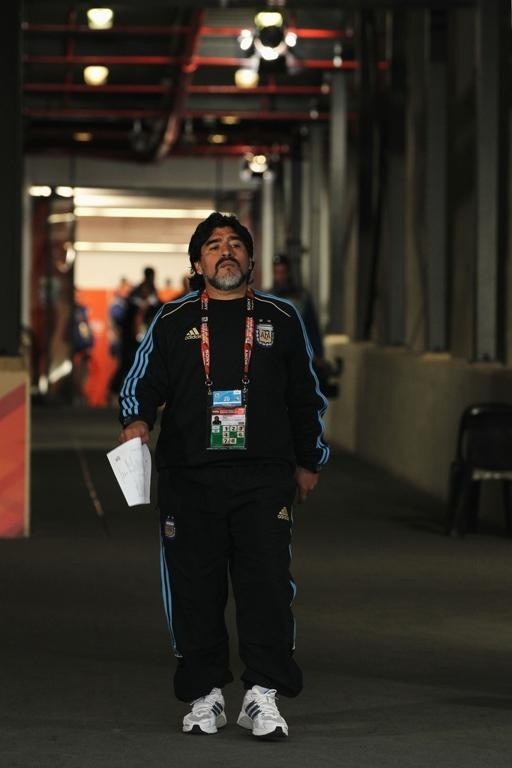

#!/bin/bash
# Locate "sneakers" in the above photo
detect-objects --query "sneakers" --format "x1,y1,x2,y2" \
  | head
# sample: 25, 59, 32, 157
184, 686, 227, 733
237, 686, 288, 738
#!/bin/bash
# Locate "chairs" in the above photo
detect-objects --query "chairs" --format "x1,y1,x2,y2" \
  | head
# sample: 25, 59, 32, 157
445, 402, 512, 536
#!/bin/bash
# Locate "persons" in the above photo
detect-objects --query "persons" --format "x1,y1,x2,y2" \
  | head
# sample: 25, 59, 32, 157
104, 279, 134, 404
262, 253, 327, 389
116, 212, 332, 740
119, 268, 162, 391
